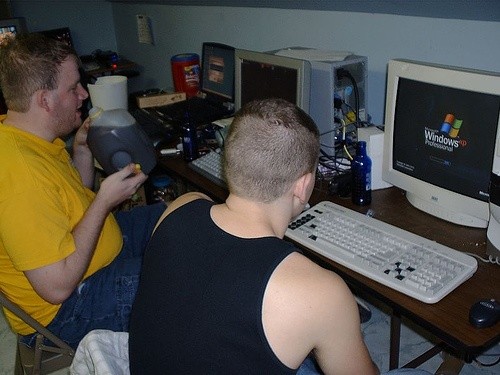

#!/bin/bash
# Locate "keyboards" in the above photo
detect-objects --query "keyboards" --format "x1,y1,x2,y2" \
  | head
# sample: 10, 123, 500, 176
285, 201, 478, 304
188, 151, 229, 192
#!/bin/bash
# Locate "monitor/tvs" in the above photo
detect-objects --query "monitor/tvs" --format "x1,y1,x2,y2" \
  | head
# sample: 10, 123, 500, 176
234, 49, 311, 116
199, 42, 236, 102
0, 15, 28, 42
381, 57, 500, 229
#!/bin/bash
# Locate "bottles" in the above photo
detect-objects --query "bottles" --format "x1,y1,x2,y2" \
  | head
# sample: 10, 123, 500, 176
87, 107, 158, 180
352, 140, 372, 207
181, 108, 199, 161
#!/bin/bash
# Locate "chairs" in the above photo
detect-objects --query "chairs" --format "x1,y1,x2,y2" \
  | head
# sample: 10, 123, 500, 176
0, 293, 75, 375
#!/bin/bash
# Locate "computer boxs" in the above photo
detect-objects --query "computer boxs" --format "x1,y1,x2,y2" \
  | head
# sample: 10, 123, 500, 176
263, 46, 368, 159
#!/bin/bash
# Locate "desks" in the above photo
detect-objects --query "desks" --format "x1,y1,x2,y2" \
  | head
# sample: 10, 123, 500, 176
146, 145, 500, 375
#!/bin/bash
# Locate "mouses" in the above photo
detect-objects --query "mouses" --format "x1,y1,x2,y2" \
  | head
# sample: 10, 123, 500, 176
469, 298, 500, 327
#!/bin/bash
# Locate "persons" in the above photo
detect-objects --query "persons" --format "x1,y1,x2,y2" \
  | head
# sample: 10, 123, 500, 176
1, 33, 371, 351
128, 98, 432, 375
